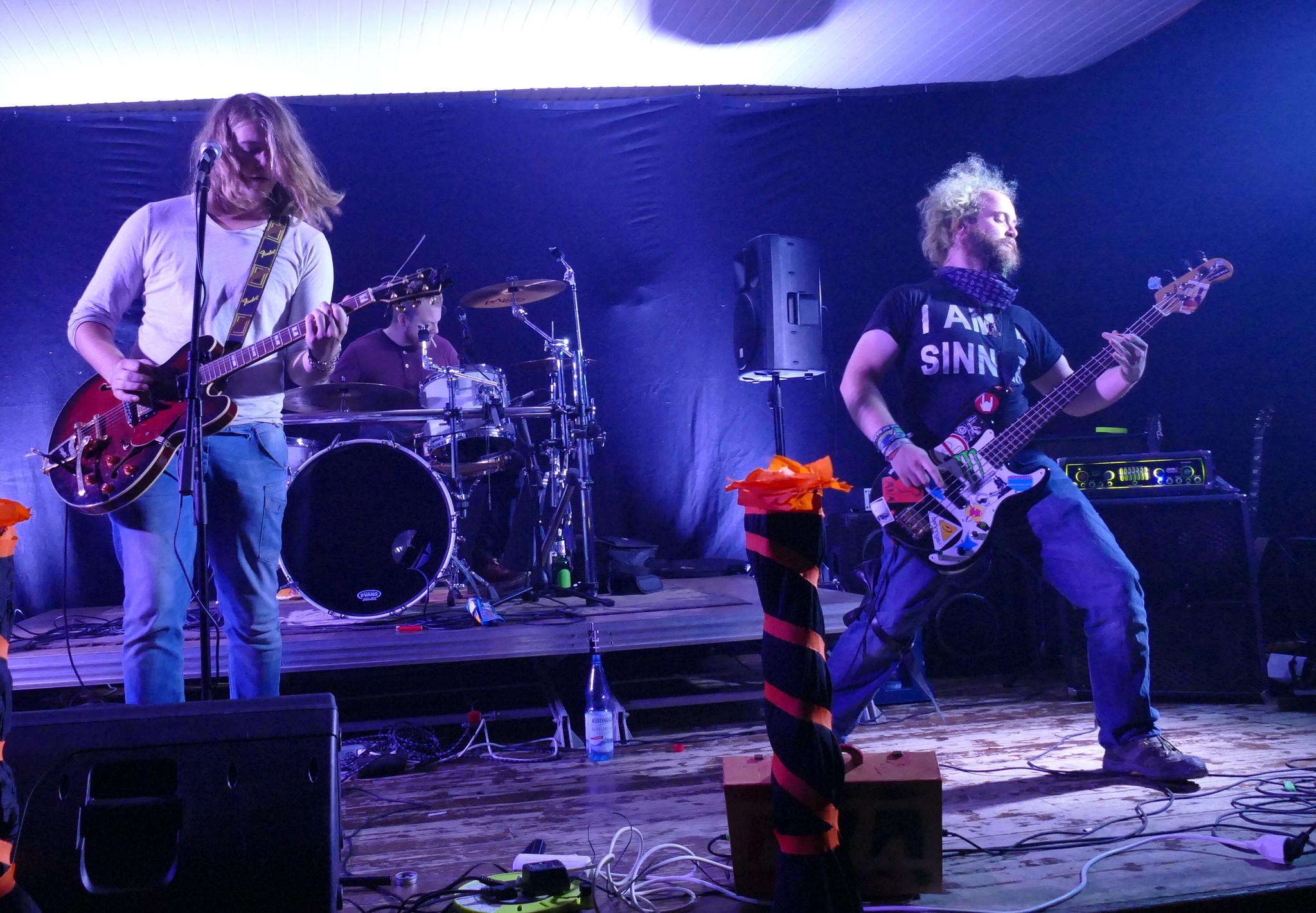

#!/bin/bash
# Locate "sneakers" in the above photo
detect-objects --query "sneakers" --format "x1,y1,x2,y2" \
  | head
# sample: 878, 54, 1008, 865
467, 558, 530, 594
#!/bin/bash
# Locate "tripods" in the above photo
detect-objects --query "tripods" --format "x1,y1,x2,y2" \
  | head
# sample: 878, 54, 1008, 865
422, 461, 616, 608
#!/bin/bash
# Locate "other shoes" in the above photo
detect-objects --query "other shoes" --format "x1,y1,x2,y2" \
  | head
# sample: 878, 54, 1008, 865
1100, 729, 1208, 782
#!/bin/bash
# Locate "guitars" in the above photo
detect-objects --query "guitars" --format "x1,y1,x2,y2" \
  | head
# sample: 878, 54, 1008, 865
47, 262, 455, 515
869, 250, 1234, 572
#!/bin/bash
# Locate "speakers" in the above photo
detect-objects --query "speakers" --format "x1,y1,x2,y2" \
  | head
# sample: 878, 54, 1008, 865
922, 546, 1059, 676
3, 691, 343, 913
842, 512, 885, 598
721, 750, 943, 897
734, 234, 827, 383
1056, 472, 1270, 705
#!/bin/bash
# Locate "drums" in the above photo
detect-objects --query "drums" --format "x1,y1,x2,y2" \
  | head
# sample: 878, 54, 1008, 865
418, 361, 517, 460
279, 438, 456, 621
283, 435, 322, 486
414, 427, 506, 475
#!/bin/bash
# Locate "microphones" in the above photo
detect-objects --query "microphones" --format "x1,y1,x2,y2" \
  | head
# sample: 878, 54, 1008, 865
459, 306, 470, 339
195, 141, 222, 193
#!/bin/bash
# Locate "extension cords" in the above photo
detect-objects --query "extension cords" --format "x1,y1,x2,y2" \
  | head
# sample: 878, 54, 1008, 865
512, 854, 593, 878
451, 871, 595, 913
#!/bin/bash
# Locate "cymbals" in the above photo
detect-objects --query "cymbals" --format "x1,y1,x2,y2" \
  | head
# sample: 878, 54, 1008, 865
462, 278, 568, 310
280, 382, 415, 413
520, 356, 599, 370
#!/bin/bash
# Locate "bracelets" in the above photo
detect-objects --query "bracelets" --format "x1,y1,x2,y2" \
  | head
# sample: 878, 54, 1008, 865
307, 347, 342, 372
873, 423, 914, 460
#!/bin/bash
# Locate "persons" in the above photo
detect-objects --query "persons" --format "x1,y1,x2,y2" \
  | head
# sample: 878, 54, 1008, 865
64, 93, 349, 707
331, 276, 531, 596
825, 155, 1211, 784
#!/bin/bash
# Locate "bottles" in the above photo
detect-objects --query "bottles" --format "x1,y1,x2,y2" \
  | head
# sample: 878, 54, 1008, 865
585, 630, 614, 761
393, 871, 419, 913
